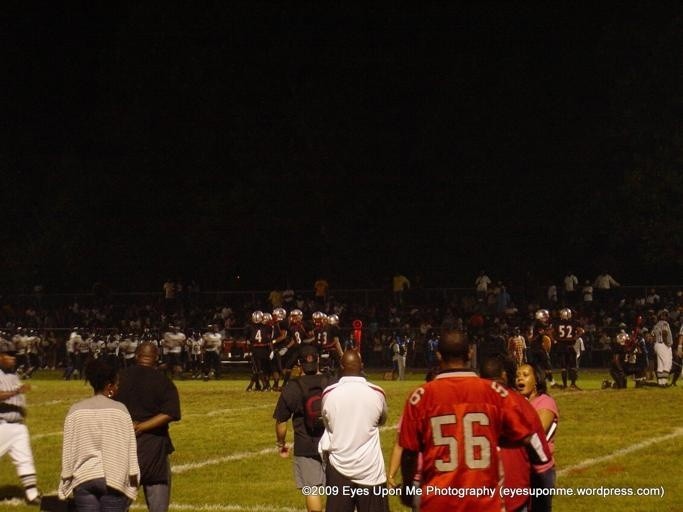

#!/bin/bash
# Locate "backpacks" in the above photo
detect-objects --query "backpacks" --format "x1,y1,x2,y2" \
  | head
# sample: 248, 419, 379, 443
293, 374, 331, 436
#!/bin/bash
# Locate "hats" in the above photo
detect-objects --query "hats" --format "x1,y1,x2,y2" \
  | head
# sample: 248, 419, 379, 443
0, 338, 19, 353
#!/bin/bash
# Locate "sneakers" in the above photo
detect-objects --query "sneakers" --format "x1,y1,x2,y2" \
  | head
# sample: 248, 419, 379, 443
550, 382, 583, 391
601, 380, 612, 390
25, 495, 42, 506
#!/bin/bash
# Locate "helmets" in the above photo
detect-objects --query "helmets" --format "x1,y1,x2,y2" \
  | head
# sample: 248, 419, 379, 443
250, 307, 340, 325
535, 309, 550, 322
559, 308, 572, 321
616, 332, 630, 346
